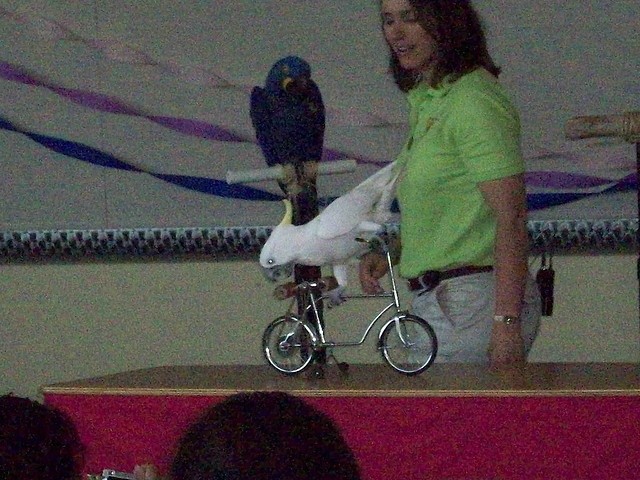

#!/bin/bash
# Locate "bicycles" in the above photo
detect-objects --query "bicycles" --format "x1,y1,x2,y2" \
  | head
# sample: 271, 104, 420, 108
262, 235, 437, 376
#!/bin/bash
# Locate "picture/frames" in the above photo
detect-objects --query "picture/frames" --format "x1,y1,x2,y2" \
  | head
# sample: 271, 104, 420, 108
2, 2, 638, 255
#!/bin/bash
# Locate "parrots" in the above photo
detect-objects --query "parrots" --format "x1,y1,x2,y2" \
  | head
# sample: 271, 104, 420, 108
258, 158, 398, 310
248, 56, 326, 370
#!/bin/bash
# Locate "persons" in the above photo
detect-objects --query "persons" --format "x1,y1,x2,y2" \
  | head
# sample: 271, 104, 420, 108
359, 0, 542, 365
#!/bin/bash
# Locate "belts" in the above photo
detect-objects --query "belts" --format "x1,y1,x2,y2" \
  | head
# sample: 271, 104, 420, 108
408, 264, 492, 295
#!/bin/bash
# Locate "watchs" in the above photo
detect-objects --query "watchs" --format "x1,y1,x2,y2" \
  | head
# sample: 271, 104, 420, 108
493, 315, 520, 326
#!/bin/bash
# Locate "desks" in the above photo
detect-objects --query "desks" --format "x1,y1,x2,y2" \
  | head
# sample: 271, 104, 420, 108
40, 361, 639, 480
